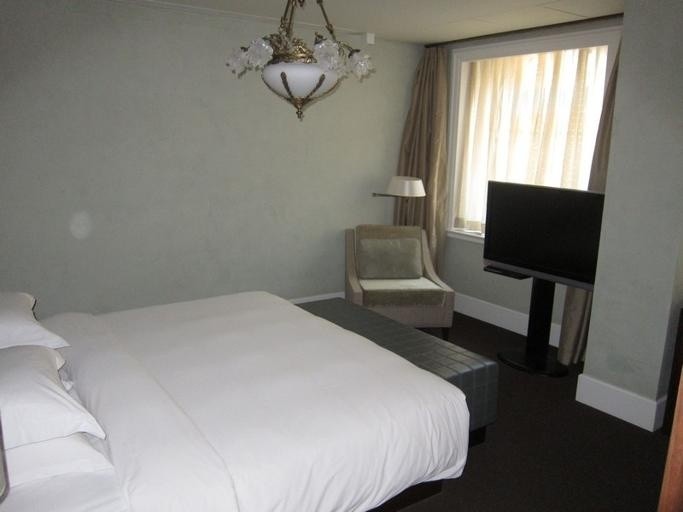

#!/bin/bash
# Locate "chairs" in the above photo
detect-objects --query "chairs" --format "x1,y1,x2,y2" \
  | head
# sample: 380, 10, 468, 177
344, 224, 455, 341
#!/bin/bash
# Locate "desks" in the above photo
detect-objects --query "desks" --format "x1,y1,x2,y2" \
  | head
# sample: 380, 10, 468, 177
481, 267, 580, 380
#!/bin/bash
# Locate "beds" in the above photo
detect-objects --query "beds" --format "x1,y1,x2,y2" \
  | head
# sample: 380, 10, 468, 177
1, 290, 472, 511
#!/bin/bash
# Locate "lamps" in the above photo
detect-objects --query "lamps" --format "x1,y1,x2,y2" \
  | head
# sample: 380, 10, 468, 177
387, 176, 427, 226
224, 0, 376, 121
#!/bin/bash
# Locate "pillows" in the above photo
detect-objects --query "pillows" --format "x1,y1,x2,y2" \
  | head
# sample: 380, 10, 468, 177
1, 368, 115, 495
355, 239, 423, 280
1, 345, 106, 451
0, 293, 68, 349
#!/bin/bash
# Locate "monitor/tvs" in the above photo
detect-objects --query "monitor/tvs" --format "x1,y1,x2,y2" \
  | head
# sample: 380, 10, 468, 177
482, 180, 605, 292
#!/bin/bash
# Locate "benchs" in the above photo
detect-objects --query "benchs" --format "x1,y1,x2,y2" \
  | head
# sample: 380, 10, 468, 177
295, 295, 499, 449
